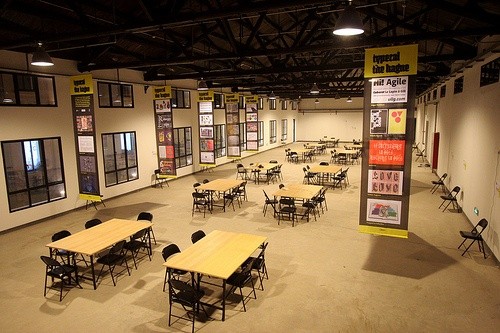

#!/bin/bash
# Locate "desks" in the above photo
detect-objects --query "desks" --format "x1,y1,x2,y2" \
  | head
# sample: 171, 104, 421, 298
193, 180, 246, 215
46, 219, 150, 290
335, 150, 358, 165
287, 149, 312, 162
272, 185, 325, 221
306, 144, 324, 152
163, 231, 268, 322
243, 164, 279, 184
350, 145, 362, 150
307, 167, 343, 189
322, 139, 336, 146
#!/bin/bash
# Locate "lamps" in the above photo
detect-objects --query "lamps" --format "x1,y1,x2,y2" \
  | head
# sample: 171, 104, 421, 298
31, 0, 366, 103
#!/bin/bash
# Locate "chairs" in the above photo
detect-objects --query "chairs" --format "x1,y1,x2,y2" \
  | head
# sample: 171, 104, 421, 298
412, 143, 425, 161
457, 218, 489, 259
430, 173, 447, 193
439, 186, 461, 212
41, 136, 364, 333
